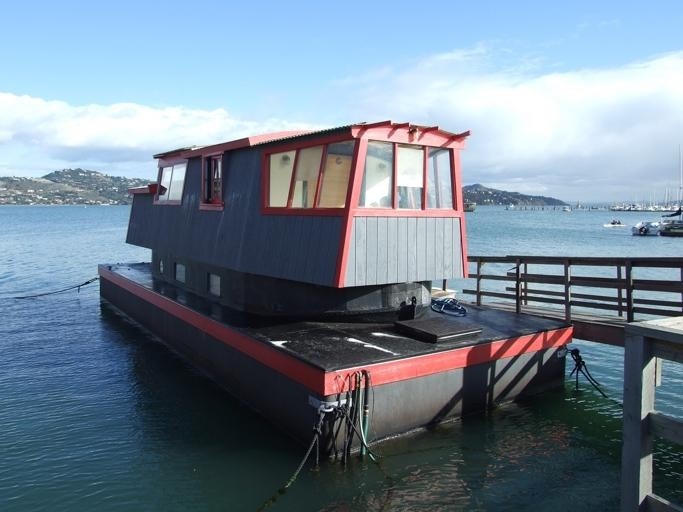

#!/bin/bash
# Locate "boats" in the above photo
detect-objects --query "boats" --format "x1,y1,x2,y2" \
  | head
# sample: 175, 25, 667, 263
463, 199, 476, 212
562, 206, 570, 212
632, 221, 664, 236
602, 220, 627, 228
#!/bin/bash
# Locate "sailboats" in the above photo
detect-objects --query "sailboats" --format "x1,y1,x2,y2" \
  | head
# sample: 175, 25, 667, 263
610, 188, 683, 211
660, 206, 683, 228
